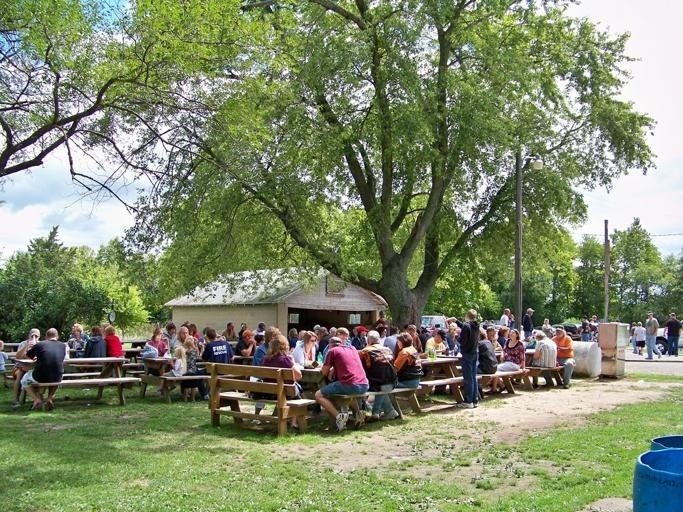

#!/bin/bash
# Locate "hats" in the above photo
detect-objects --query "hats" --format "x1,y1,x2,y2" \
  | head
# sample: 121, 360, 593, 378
647, 311, 653, 316
668, 312, 675, 316
379, 310, 386, 315
242, 329, 256, 339
29, 328, 40, 338
527, 307, 535, 313
356, 325, 369, 335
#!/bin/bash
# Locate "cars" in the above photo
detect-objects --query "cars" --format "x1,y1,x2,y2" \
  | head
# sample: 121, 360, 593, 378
420, 313, 587, 341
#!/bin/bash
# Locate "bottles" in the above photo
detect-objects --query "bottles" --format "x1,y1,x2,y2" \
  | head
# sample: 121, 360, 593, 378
74, 339, 87, 350
317, 351, 323, 367
164, 349, 176, 358
30, 336, 36, 346
425, 344, 459, 359
195, 345, 205, 356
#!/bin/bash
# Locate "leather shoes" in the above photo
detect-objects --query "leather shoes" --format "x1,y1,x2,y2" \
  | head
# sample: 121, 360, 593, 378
291, 421, 298, 430
483, 385, 506, 395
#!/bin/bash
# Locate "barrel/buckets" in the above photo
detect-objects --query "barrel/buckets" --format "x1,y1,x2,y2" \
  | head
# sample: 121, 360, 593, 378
651, 435, 683, 450
632, 448, 683, 512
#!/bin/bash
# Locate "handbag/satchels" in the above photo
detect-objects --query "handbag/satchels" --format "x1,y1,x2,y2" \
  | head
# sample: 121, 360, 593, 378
142, 343, 158, 359
252, 379, 262, 400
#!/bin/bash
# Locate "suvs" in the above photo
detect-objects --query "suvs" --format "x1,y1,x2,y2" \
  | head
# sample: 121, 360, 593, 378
652, 319, 682, 356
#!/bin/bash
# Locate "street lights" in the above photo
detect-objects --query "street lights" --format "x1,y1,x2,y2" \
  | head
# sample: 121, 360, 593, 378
514, 153, 543, 334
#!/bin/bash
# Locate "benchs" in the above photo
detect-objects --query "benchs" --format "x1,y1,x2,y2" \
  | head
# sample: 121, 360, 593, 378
206, 363, 317, 437
2, 341, 255, 410
436, 346, 576, 403
300, 353, 484, 433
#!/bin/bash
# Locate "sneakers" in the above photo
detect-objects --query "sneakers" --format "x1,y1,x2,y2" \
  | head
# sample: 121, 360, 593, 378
353, 410, 366, 430
335, 412, 348, 433
380, 409, 400, 421
204, 394, 209, 400
474, 400, 480, 407
541, 383, 554, 388
455, 402, 474, 409
10, 399, 22, 409
366, 413, 379, 422
564, 384, 569, 389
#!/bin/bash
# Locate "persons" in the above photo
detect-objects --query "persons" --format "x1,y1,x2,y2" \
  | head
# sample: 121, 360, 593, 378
0, 339, 10, 390
18, 326, 66, 411
67, 322, 125, 378
8, 327, 41, 409
